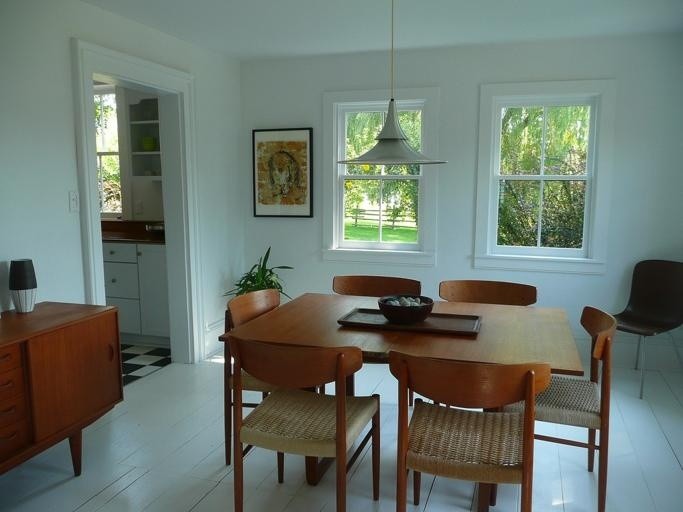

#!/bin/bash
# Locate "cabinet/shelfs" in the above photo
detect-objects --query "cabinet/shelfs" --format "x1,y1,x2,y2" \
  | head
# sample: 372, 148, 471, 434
104, 241, 166, 345
0, 301, 125, 477
116, 86, 164, 220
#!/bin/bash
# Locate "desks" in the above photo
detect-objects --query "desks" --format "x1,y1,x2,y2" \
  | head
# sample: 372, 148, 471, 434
221, 293, 584, 504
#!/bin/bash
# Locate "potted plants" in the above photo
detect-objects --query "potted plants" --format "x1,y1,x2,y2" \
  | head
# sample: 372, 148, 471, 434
223, 249, 294, 298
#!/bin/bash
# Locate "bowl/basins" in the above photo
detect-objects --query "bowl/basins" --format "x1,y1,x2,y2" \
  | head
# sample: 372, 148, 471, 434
144, 223, 164, 241
378, 294, 434, 322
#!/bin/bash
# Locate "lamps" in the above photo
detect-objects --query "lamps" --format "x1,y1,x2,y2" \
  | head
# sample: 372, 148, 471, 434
336, 0, 449, 167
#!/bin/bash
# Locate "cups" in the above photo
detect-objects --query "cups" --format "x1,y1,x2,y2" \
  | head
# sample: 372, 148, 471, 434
9, 259, 37, 290
12, 289, 37, 314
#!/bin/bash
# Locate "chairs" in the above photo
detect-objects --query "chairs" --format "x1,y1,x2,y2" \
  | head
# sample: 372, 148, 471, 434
330, 274, 538, 410
388, 349, 550, 511
228, 334, 381, 510
490, 306, 618, 510
613, 261, 682, 400
224, 288, 327, 465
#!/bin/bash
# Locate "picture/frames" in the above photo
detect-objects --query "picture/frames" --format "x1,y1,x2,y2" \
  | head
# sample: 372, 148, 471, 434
254, 129, 312, 218
